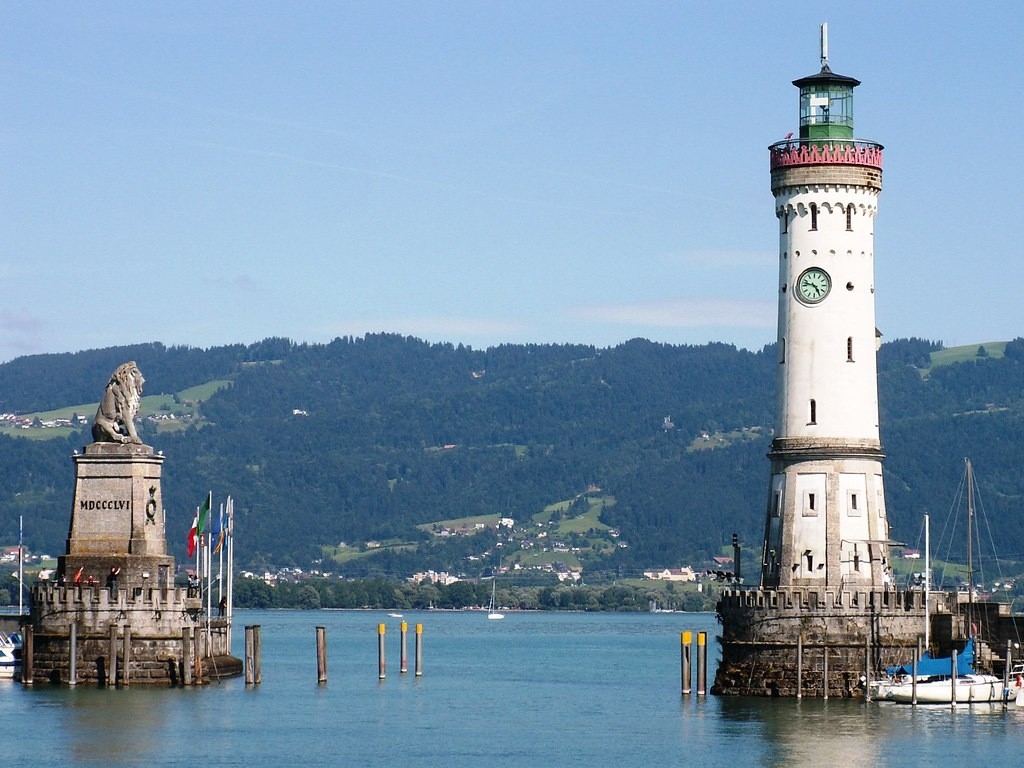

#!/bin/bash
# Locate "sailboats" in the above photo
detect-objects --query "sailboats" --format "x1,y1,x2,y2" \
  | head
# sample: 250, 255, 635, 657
857, 455, 1024, 708
487, 578, 505, 620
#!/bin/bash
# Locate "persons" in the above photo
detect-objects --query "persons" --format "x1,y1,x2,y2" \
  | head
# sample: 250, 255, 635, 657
73, 566, 84, 598
219, 596, 227, 620
188, 575, 197, 597
1015, 674, 1023, 690
106, 567, 121, 599
39, 567, 57, 586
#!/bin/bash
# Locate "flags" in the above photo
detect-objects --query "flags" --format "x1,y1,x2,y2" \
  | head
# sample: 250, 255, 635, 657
188, 494, 232, 559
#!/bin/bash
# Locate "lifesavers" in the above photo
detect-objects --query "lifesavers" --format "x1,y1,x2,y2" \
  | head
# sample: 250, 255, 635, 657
964, 622, 977, 638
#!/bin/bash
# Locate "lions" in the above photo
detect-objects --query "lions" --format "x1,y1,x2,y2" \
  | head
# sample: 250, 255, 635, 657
92, 360, 146, 445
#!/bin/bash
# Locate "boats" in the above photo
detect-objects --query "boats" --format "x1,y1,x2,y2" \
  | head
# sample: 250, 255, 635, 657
0, 628, 23, 680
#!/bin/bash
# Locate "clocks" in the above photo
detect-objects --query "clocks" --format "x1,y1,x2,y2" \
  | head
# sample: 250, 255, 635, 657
796, 268, 832, 304
